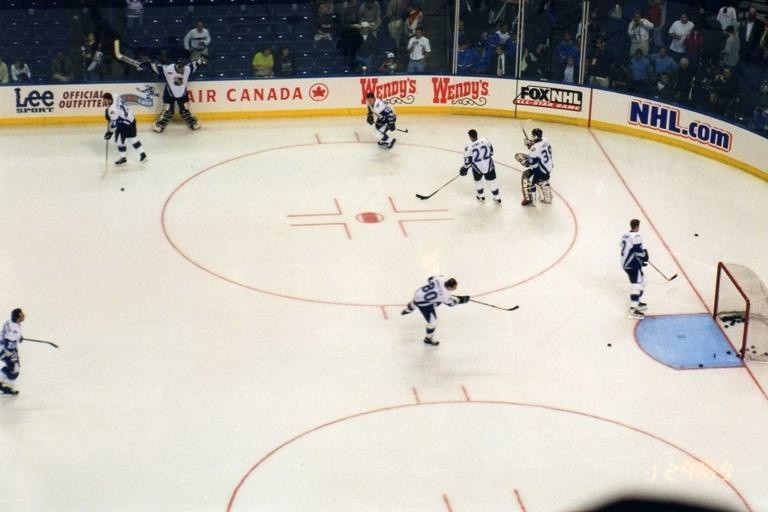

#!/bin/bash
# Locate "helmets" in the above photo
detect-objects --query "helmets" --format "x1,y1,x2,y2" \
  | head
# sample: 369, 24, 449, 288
532, 128, 542, 143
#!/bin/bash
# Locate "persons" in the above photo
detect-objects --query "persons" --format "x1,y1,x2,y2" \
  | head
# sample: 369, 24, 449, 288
620, 219, 648, 320
101, 91, 145, 166
459, 129, 500, 205
0, 308, 25, 396
364, 91, 396, 148
1, 0, 768, 134
516, 128, 553, 206
400, 275, 470, 346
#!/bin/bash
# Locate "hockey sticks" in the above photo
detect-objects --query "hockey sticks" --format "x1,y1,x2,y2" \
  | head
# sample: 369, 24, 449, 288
521, 119, 531, 139
647, 260, 676, 282
114, 39, 140, 68
373, 121, 408, 133
22, 338, 59, 348
99, 121, 108, 182
416, 165, 472, 199
451, 294, 519, 311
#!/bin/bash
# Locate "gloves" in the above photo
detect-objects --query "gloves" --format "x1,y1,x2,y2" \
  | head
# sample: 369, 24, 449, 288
459, 166, 469, 176
104, 132, 113, 140
457, 296, 470, 303
389, 122, 395, 131
367, 116, 375, 124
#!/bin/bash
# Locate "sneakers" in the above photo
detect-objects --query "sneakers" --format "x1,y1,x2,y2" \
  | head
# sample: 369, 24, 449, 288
377, 138, 395, 149
114, 152, 148, 165
628, 302, 647, 317
0, 382, 20, 395
476, 196, 501, 204
423, 337, 440, 346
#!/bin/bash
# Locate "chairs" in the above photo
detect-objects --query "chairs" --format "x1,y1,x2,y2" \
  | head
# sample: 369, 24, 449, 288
0, 0, 753, 133
